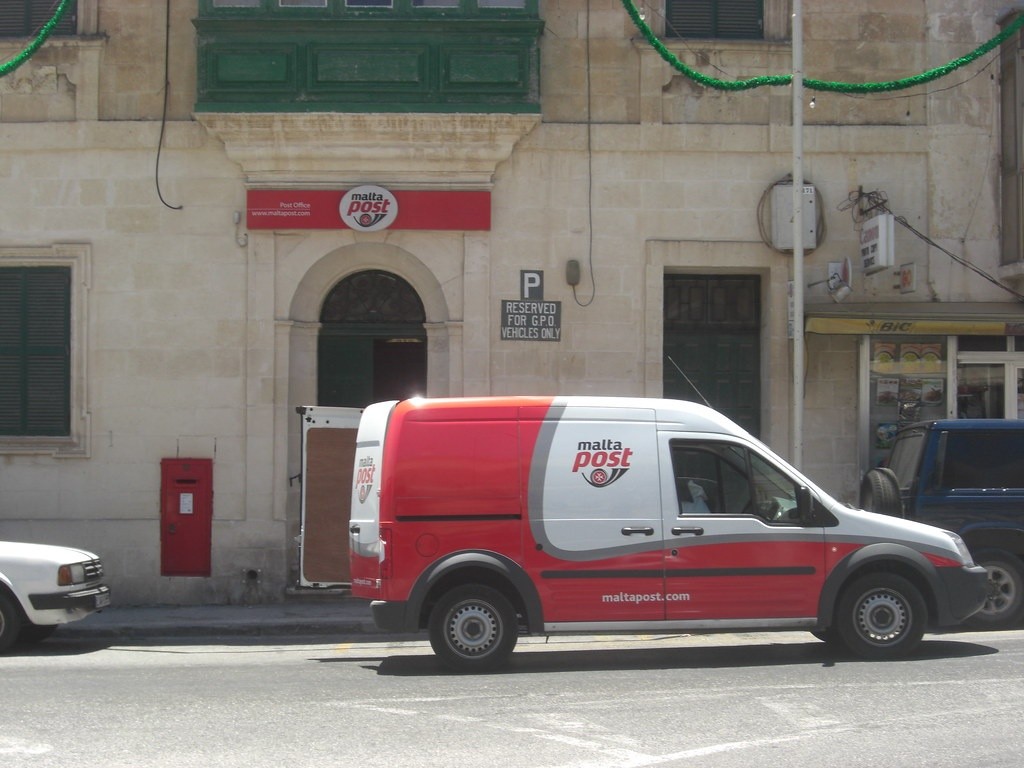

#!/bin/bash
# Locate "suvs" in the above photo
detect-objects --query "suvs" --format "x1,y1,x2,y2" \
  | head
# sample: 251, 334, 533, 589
855, 417, 1022, 627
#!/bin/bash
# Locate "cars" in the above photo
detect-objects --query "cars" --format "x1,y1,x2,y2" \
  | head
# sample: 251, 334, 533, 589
0, 539, 109, 653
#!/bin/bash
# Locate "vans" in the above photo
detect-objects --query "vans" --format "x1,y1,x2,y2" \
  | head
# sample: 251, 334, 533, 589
293, 393, 1010, 661
